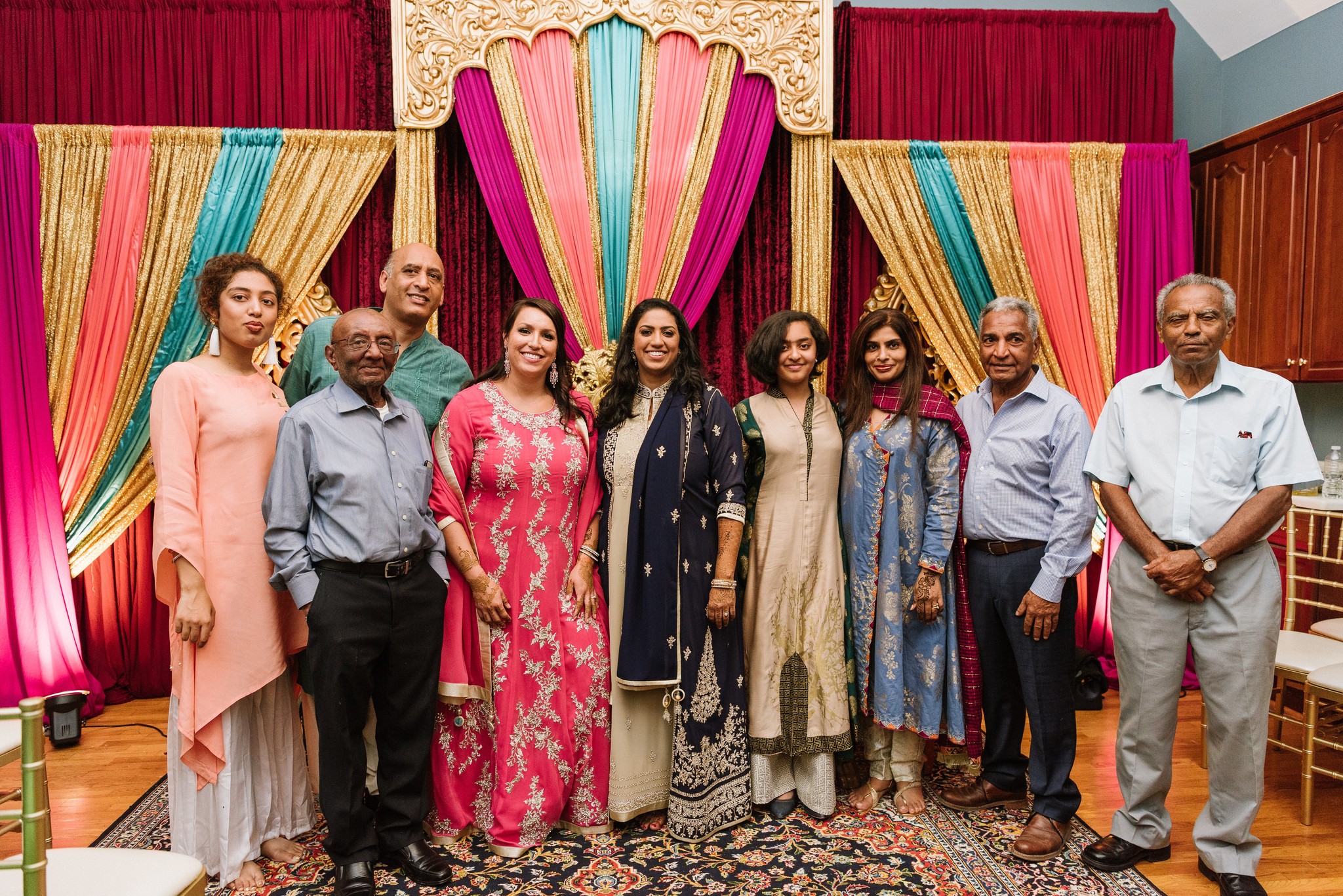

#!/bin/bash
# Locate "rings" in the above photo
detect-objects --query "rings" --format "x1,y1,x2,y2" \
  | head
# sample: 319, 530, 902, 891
932, 603, 938, 607
591, 595, 596, 599
932, 606, 939, 609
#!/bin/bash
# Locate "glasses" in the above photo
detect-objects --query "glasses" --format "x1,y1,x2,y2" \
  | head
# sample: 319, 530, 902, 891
332, 336, 402, 355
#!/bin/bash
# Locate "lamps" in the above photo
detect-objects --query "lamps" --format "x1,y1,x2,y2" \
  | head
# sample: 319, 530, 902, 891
44, 690, 90, 747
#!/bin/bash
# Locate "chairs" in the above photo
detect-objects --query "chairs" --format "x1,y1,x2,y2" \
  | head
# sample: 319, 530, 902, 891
0, 707, 53, 850
1200, 505, 1343, 827
0, 695, 206, 896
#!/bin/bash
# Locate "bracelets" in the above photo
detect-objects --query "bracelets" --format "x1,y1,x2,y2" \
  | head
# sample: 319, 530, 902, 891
172, 554, 181, 564
710, 579, 737, 589
170, 550, 174, 553
578, 549, 598, 562
581, 544, 599, 557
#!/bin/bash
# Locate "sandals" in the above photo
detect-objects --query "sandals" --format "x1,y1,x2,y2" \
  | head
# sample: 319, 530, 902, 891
894, 780, 926, 817
848, 777, 893, 813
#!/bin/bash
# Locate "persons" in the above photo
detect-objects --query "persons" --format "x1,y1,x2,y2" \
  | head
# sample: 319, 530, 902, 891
149, 255, 311, 891
832, 307, 981, 815
588, 298, 753, 842
429, 299, 610, 858
732, 312, 863, 819
936, 298, 1097, 861
1081, 274, 1324, 896
278, 244, 475, 796
261, 307, 452, 896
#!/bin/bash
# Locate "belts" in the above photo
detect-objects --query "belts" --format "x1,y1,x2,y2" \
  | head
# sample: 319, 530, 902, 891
318, 549, 426, 578
966, 538, 1048, 556
1162, 540, 1243, 560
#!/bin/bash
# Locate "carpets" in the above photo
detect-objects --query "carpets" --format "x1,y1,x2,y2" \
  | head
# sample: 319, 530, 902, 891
89, 734, 1170, 896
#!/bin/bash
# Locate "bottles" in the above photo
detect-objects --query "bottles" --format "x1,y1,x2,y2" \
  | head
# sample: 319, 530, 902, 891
1322, 446, 1343, 500
1291, 486, 1318, 495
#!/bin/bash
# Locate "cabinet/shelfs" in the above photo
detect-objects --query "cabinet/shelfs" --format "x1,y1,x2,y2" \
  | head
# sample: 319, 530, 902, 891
1266, 511, 1343, 633
1189, 109, 1343, 384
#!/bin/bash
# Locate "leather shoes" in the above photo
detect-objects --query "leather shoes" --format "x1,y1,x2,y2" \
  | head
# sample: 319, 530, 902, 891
939, 772, 1028, 810
1080, 832, 1171, 870
333, 858, 376, 895
1198, 853, 1267, 896
397, 838, 453, 884
1015, 813, 1072, 860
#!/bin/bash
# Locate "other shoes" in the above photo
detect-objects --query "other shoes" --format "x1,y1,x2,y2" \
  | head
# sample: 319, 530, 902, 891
770, 795, 795, 818
804, 800, 826, 819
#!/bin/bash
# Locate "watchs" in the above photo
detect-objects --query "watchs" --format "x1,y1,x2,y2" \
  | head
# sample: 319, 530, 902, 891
1192, 545, 1218, 575
722, 612, 729, 616
